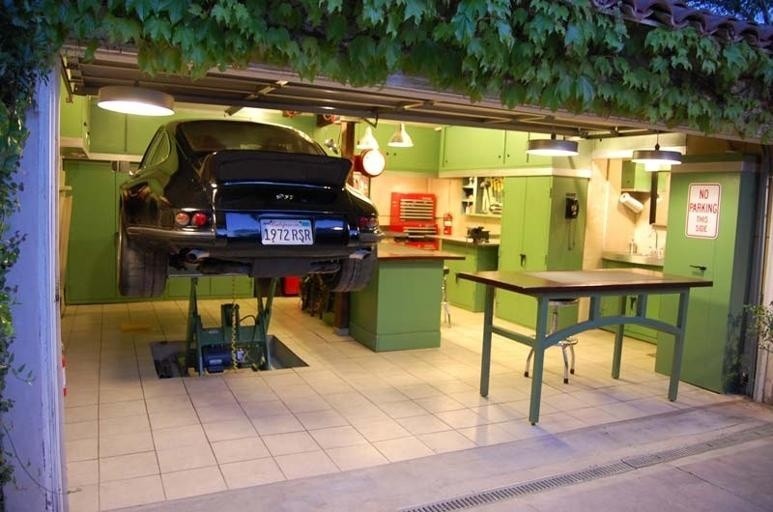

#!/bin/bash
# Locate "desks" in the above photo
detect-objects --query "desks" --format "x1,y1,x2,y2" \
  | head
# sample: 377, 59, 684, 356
456, 269, 714, 426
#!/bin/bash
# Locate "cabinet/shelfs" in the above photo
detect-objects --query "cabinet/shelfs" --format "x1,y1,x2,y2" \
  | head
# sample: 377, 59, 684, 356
621, 157, 665, 193
345, 113, 438, 176
598, 258, 663, 344
438, 124, 592, 177
494, 175, 588, 328
60, 93, 343, 307
460, 176, 501, 217
350, 246, 466, 353
441, 238, 499, 313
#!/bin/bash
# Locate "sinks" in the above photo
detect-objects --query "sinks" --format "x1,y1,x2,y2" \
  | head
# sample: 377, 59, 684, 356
627, 256, 659, 264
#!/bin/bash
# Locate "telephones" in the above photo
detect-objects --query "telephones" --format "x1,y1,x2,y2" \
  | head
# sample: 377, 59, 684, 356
565, 198, 579, 218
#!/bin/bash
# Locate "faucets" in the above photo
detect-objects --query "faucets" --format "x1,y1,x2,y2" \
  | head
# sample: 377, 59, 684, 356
649, 230, 659, 253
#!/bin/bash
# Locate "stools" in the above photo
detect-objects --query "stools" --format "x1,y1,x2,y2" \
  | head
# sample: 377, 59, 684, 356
525, 298, 579, 383
441, 265, 452, 325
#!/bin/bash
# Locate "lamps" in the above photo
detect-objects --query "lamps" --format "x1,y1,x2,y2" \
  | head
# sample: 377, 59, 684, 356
359, 126, 380, 149
631, 133, 683, 164
96, 86, 176, 117
386, 122, 413, 147
527, 132, 578, 156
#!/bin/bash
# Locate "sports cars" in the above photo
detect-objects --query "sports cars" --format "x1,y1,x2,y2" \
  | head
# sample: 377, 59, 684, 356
109, 118, 384, 297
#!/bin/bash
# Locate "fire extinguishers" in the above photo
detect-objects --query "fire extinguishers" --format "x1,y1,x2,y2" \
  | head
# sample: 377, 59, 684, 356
443, 210, 453, 235
